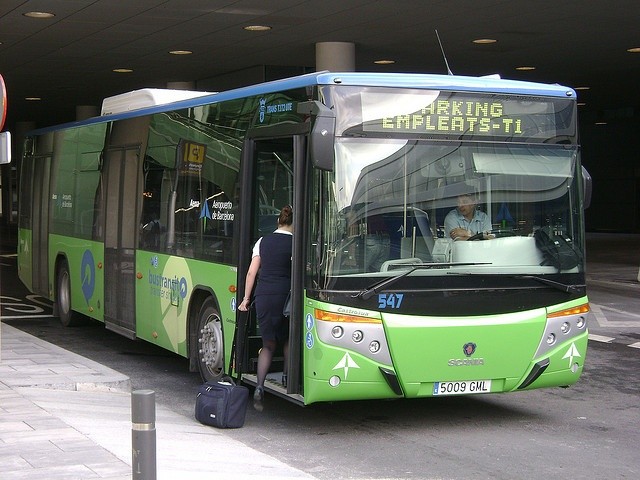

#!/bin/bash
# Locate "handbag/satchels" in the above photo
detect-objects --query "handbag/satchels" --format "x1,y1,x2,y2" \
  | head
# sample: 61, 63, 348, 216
283, 290, 291, 317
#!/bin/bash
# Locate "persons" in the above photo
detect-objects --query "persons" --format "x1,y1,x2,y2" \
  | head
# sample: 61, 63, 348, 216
444, 187, 493, 244
359, 199, 392, 272
342, 195, 359, 268
235, 206, 297, 412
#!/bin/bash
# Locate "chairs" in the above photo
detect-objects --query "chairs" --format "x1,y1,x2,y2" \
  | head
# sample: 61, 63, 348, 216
436, 182, 465, 237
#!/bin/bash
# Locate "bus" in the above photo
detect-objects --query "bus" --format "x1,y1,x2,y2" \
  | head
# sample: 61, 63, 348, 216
17, 30, 593, 406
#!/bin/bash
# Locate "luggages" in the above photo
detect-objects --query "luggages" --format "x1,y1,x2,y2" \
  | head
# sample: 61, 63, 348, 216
195, 306, 251, 428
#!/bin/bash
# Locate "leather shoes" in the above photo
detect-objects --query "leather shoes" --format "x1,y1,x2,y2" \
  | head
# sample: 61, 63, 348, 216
282, 375, 287, 387
253, 386, 264, 413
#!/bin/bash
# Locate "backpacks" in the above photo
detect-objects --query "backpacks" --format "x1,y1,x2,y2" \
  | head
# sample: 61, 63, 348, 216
533, 225, 582, 273
